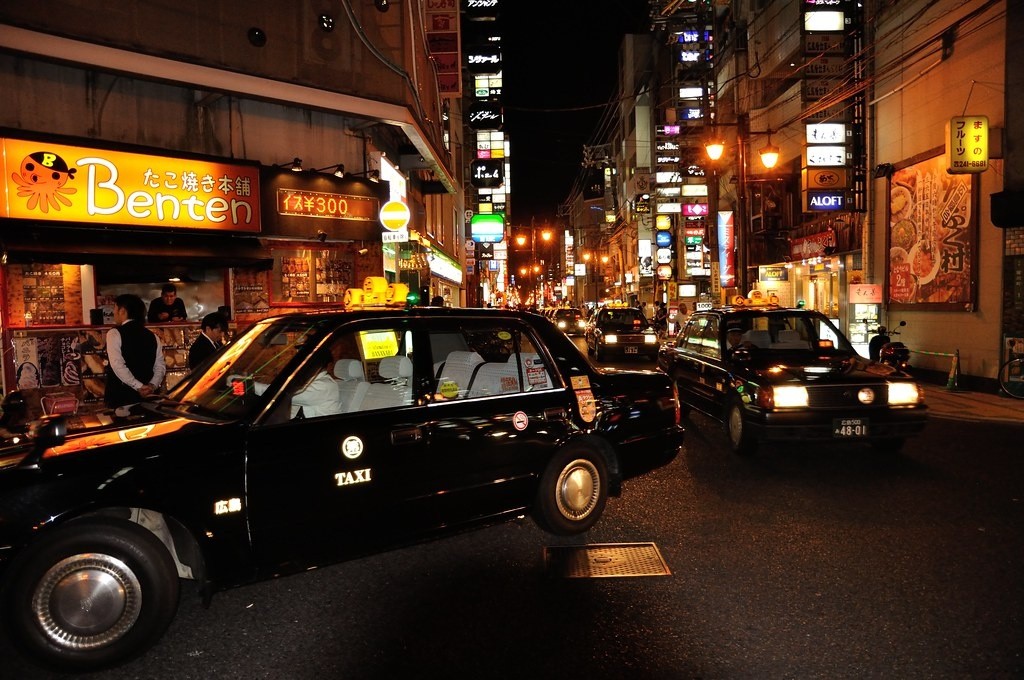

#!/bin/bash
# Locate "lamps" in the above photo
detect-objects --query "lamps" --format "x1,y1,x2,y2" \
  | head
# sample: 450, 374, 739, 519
318, 233, 327, 242
310, 164, 344, 178
273, 158, 302, 171
346, 169, 379, 183
874, 163, 892, 178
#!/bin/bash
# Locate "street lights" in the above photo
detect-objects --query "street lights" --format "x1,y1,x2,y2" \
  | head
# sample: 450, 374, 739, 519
519, 263, 542, 308
517, 216, 552, 311
702, 111, 780, 299
583, 245, 608, 311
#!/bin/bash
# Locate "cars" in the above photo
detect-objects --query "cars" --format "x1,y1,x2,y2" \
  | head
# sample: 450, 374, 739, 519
584, 308, 660, 362
539, 307, 585, 336
659, 290, 931, 464
1, 304, 688, 672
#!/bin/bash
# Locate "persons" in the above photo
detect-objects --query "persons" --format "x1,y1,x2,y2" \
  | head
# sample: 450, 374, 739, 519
634, 300, 697, 340
768, 296, 786, 343
226, 335, 340, 419
434, 296, 444, 306
148, 284, 187, 323
529, 305, 534, 313
869, 326, 891, 361
189, 312, 231, 391
475, 298, 484, 308
105, 293, 166, 407
726, 324, 753, 356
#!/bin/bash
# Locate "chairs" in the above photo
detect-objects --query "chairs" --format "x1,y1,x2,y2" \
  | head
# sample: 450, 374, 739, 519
748, 330, 771, 348
334, 359, 371, 412
360, 356, 413, 411
625, 316, 634, 324
769, 330, 809, 349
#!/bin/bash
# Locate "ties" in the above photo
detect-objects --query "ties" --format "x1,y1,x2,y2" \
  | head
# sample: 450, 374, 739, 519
214, 342, 219, 349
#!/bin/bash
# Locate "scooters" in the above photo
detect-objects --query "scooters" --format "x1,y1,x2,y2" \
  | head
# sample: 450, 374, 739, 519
861, 316, 911, 371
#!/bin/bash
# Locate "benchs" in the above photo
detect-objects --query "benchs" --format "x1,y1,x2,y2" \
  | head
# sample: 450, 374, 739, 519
434, 350, 553, 398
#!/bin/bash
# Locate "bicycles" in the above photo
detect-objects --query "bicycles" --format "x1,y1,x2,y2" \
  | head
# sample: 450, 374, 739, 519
999, 337, 1024, 400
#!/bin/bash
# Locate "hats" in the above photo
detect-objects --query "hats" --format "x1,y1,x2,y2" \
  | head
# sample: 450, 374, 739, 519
728, 323, 742, 332
679, 303, 685, 309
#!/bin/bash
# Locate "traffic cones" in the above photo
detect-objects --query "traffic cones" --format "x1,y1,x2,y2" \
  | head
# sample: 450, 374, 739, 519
947, 349, 962, 391
